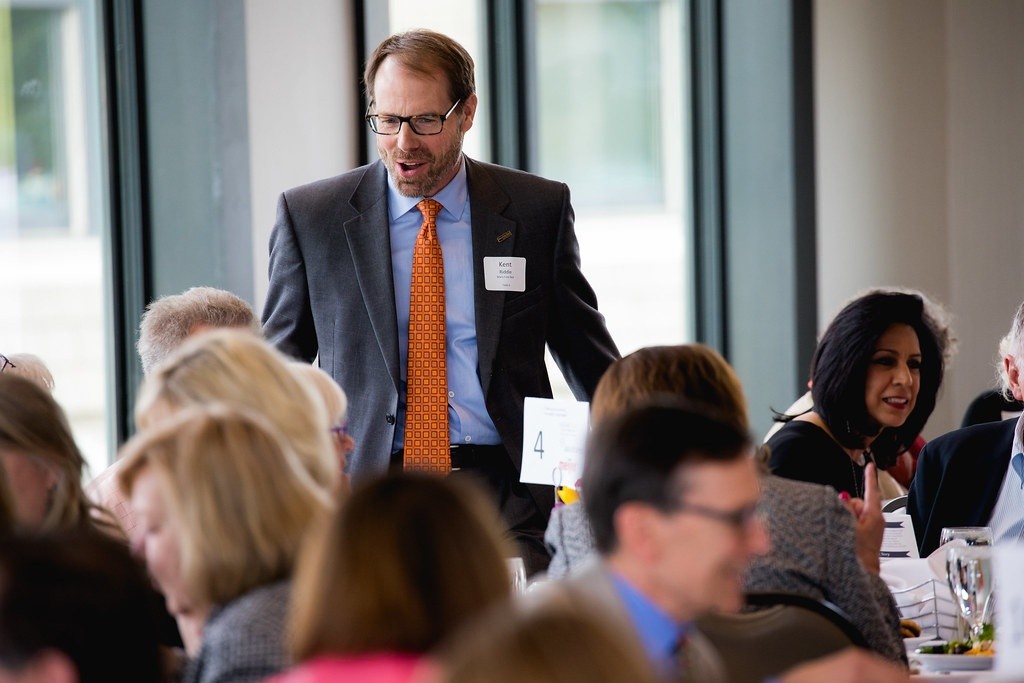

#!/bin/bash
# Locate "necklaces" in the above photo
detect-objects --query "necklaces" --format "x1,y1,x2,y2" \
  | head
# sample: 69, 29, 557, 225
846, 420, 869, 501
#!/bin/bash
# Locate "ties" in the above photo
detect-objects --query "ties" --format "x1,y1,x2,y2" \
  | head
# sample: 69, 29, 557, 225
403, 199, 452, 476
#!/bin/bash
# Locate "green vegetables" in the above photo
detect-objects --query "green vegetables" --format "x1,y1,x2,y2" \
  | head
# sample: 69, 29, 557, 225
920, 621, 994, 655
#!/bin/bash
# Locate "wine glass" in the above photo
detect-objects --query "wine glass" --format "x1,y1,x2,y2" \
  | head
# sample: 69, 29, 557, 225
945, 545, 994, 649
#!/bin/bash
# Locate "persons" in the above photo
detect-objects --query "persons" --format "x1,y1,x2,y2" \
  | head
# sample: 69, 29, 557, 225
0, 286, 1024, 683
258, 29, 623, 580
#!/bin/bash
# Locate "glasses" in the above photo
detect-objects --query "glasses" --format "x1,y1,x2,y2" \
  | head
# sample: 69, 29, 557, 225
329, 419, 349, 440
668, 497, 768, 537
0, 354, 16, 377
365, 99, 462, 134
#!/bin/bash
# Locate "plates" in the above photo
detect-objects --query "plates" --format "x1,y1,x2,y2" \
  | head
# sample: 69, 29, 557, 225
908, 652, 992, 672
903, 633, 937, 652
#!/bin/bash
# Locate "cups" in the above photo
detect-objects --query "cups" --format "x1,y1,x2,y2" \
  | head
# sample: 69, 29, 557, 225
939, 527, 993, 548
